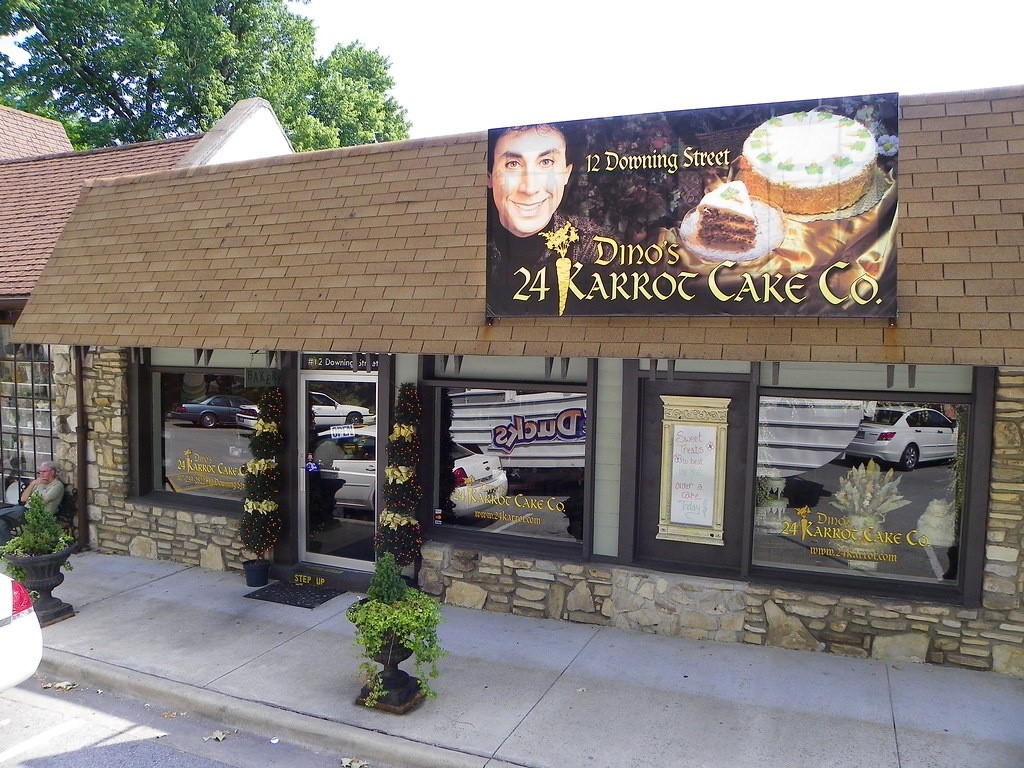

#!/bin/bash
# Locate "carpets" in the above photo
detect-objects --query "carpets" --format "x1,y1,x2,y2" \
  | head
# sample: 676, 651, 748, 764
243, 580, 347, 609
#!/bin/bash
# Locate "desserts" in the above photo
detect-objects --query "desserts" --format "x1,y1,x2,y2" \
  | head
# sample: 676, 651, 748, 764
701, 182, 756, 249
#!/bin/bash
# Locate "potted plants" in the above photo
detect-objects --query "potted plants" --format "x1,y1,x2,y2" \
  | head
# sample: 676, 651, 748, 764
829, 457, 913, 564
346, 550, 450, 717
0, 489, 76, 629
372, 381, 425, 587
233, 385, 289, 587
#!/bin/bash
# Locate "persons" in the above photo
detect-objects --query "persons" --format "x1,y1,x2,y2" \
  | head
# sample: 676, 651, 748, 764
0, 460, 64, 559
488, 121, 632, 275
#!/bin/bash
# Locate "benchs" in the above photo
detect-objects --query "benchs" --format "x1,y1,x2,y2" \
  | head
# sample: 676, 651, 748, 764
1, 483, 78, 544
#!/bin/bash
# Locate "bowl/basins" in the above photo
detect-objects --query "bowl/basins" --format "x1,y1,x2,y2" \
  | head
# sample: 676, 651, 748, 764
37, 403, 49, 409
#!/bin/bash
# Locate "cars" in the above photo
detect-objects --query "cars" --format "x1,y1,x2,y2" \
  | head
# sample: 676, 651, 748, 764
318, 428, 508, 522
171, 395, 257, 428
846, 406, 958, 471
236, 391, 374, 428
0, 571, 44, 693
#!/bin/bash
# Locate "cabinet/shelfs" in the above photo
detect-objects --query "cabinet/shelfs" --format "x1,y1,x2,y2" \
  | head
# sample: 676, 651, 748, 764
0, 361, 57, 480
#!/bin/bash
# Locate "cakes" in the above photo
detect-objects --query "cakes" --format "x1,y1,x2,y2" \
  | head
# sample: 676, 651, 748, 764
741, 112, 879, 214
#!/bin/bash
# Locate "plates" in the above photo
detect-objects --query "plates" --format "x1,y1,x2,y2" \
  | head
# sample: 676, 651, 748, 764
680, 195, 790, 262
